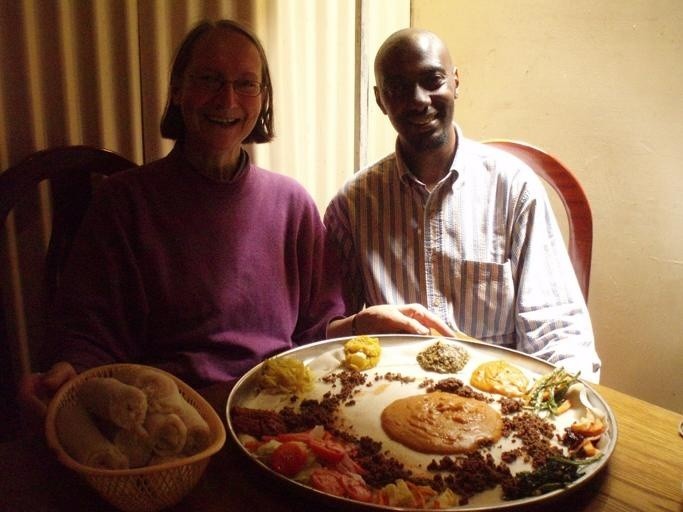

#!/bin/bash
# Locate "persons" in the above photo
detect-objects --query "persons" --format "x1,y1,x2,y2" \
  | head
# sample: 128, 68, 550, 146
323, 28, 602, 387
17, 18, 459, 443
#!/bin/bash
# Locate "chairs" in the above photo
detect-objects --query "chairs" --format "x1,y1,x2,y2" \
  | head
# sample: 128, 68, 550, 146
0, 142, 141, 395
478, 137, 595, 323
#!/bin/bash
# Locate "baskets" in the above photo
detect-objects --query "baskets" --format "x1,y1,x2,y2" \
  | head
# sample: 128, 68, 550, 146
46, 364, 229, 511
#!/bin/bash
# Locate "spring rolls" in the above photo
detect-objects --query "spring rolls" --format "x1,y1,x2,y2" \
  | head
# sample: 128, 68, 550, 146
55, 366, 210, 505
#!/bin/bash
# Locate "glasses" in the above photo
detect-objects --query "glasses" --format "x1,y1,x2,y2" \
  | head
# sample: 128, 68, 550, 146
189, 68, 265, 97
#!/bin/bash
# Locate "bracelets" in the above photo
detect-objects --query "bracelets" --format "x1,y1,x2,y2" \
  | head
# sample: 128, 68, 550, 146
351, 314, 357, 336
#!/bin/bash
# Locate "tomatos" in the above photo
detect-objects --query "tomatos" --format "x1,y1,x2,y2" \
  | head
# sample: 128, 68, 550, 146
275, 438, 374, 501
